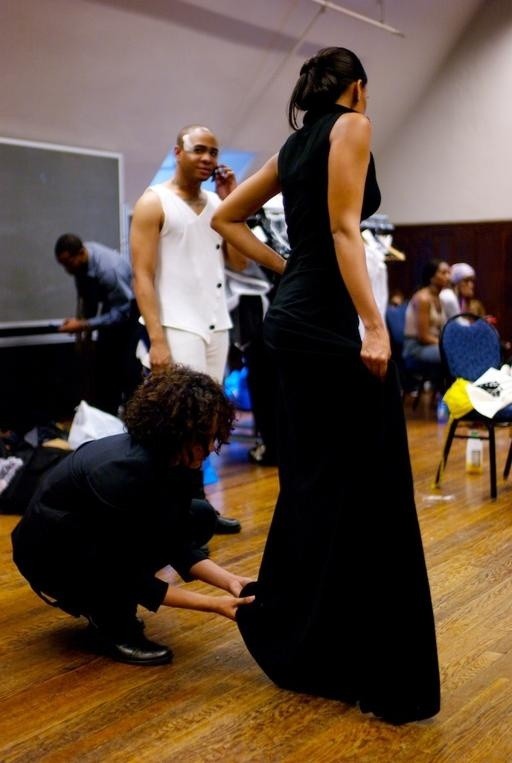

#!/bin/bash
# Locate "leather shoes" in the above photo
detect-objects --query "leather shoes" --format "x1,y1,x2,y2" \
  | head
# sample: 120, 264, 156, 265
88, 617, 172, 664
201, 517, 240, 554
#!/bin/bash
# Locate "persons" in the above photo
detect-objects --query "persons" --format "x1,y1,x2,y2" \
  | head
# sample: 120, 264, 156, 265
438, 260, 478, 326
10, 361, 261, 673
208, 45, 444, 728
402, 256, 454, 377
127, 123, 253, 389
52, 231, 142, 418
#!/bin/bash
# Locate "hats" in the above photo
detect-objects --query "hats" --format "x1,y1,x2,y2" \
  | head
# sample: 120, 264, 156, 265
450, 263, 475, 284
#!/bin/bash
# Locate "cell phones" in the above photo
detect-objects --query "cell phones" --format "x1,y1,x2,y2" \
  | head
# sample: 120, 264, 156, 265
47, 323, 63, 328
213, 166, 227, 180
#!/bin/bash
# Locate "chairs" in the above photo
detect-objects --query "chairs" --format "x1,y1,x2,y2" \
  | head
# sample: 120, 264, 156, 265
383, 303, 511, 499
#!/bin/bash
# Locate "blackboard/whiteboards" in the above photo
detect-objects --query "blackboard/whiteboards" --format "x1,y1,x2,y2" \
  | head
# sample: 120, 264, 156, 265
0, 137, 133, 330
